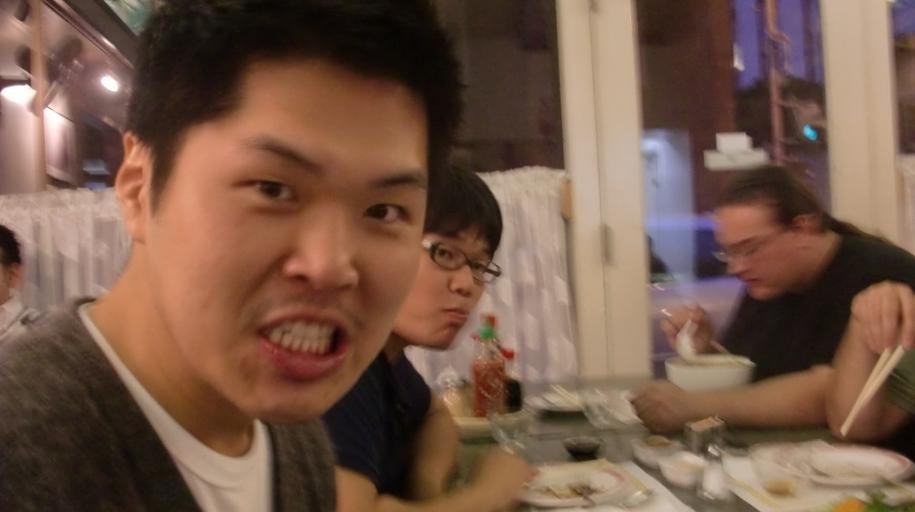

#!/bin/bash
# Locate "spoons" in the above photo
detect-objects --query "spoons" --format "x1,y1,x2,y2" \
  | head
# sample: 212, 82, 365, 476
672, 318, 699, 365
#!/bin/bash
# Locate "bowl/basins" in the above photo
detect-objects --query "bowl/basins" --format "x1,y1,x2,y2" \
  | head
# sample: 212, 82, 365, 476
630, 432, 705, 490
660, 352, 755, 392
562, 435, 603, 458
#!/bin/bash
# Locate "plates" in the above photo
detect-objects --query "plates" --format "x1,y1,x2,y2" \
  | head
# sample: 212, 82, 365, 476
769, 439, 911, 487
516, 464, 628, 507
454, 410, 527, 439
524, 391, 593, 413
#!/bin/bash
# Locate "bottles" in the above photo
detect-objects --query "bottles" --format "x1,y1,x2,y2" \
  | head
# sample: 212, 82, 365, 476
471, 312, 521, 417
696, 442, 730, 505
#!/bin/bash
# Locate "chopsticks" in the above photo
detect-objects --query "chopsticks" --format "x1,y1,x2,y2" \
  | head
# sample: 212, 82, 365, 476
838, 327, 907, 439
550, 383, 579, 407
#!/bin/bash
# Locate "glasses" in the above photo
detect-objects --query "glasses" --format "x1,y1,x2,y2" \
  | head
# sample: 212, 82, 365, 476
708, 227, 788, 264
421, 238, 501, 283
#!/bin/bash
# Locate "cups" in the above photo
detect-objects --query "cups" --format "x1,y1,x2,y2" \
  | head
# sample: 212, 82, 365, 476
747, 440, 797, 496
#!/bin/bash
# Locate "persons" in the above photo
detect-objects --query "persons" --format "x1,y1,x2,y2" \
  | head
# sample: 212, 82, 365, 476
824, 278, 915, 444
0, 224, 53, 351
2, 0, 469, 512
630, 164, 915, 435
319, 168, 534, 511
